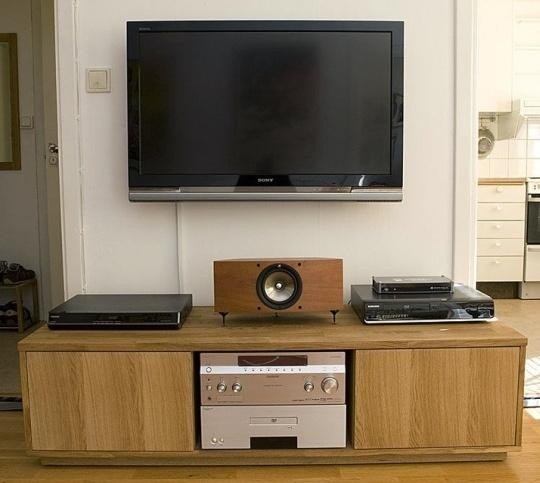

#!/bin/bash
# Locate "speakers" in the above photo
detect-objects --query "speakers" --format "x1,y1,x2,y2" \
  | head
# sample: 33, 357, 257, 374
214, 258, 343, 325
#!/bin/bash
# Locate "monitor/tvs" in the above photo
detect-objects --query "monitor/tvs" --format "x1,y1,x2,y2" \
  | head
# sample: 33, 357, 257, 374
127, 20, 404, 187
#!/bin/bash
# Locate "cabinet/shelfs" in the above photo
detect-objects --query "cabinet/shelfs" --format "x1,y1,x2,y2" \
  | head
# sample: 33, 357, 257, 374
477, 178, 526, 284
16, 306, 528, 466
